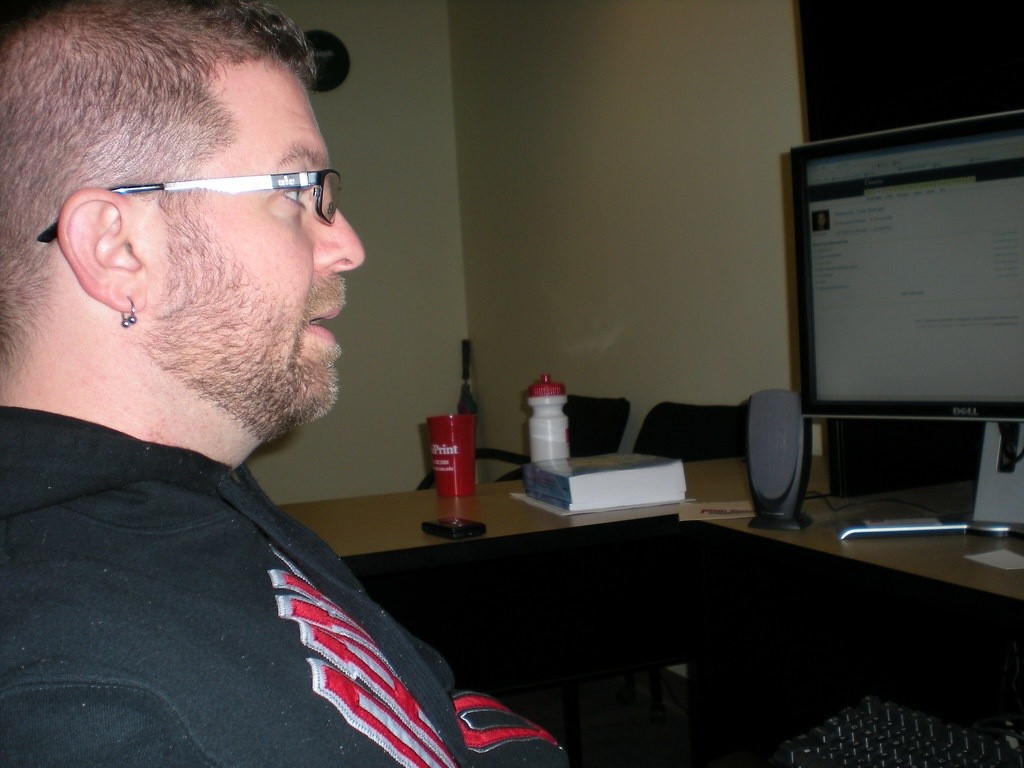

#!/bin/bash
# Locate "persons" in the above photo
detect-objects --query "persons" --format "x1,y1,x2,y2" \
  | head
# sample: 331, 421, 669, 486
0, 0, 572, 768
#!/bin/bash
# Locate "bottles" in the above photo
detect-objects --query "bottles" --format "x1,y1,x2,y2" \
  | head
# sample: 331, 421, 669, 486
526, 374, 571, 461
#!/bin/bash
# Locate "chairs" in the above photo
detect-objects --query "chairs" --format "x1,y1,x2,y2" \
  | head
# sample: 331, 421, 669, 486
414, 395, 631, 490
632, 401, 749, 463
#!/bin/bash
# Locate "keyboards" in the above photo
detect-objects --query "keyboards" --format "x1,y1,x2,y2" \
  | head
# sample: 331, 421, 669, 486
773, 695, 1024, 768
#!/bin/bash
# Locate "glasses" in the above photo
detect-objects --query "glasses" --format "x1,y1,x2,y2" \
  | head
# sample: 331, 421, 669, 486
36, 169, 341, 243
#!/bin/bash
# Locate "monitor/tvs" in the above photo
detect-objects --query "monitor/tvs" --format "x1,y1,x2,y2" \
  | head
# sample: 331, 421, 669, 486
790, 110, 1023, 541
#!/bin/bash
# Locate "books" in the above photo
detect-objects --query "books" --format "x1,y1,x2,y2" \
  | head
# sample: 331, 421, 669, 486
522, 451, 687, 511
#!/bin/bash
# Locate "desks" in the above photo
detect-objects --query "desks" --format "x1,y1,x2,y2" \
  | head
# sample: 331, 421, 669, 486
282, 459, 1023, 768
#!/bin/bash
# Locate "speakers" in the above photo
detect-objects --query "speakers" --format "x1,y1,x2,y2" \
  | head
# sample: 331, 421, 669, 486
744, 390, 813, 532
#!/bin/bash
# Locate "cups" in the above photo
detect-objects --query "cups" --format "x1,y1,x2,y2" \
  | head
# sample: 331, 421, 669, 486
427, 414, 476, 496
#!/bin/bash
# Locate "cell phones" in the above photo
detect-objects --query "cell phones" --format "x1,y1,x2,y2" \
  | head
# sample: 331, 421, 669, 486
422, 516, 487, 540
